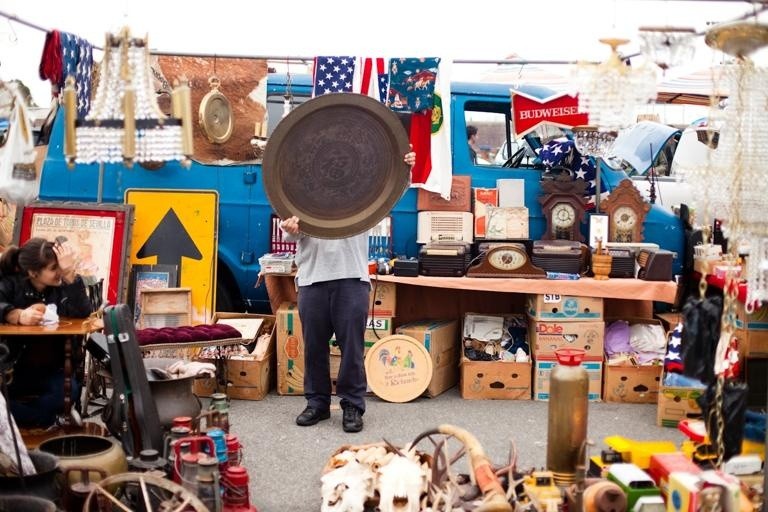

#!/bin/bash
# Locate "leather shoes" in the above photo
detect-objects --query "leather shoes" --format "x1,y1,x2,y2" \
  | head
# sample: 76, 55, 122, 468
342, 407, 362, 432
296, 407, 330, 426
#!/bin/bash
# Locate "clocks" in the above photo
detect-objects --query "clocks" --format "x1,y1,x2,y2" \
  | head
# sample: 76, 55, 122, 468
612, 205, 638, 231
488, 249, 526, 271
551, 202, 576, 229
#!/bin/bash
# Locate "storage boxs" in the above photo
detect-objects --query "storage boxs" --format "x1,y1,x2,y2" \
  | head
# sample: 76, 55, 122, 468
727, 297, 768, 359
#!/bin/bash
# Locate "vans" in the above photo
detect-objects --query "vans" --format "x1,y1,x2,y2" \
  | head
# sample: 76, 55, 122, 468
36, 60, 703, 317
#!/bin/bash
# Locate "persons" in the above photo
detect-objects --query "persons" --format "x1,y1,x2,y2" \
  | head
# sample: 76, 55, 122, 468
280, 143, 417, 433
0, 235, 92, 430
465, 124, 481, 166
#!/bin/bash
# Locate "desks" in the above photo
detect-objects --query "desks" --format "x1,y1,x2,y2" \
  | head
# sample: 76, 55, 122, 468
1, 318, 104, 433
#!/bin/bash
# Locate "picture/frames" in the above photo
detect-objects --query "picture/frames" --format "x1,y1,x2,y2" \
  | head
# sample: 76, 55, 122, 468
9, 197, 136, 305
